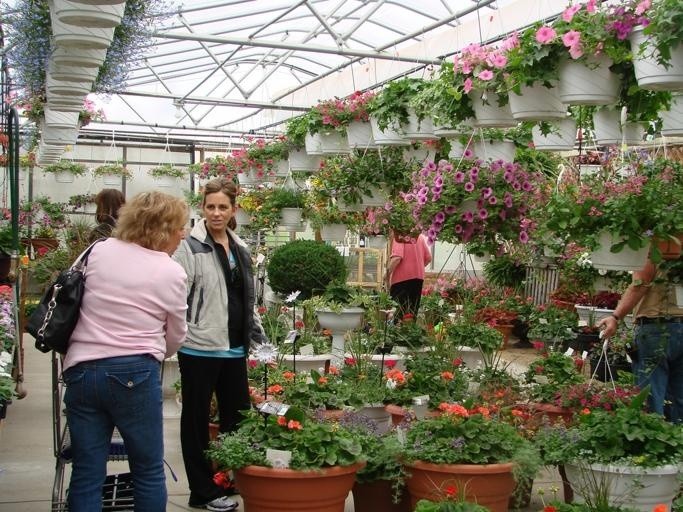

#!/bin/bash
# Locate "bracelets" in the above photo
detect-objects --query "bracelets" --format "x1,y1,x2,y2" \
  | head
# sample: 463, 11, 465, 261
610, 314, 619, 321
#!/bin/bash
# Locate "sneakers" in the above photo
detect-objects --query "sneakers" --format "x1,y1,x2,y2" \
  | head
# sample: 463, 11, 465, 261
189, 496, 239, 512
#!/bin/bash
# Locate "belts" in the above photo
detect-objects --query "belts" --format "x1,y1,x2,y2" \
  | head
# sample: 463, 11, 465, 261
635, 316, 683, 324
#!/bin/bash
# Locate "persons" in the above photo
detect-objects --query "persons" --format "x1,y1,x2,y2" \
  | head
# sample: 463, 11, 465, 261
165, 177, 257, 512
594, 234, 683, 423
58, 190, 189, 512
385, 227, 433, 326
87, 188, 125, 243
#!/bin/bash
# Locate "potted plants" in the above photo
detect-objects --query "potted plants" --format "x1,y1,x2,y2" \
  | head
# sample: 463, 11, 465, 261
179, 0, 683, 512
0, 0, 188, 436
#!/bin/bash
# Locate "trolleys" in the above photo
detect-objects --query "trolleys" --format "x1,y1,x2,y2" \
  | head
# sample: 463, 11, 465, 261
52, 347, 167, 512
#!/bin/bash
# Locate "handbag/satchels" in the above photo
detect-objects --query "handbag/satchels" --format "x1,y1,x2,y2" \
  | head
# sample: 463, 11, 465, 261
24, 270, 86, 355
